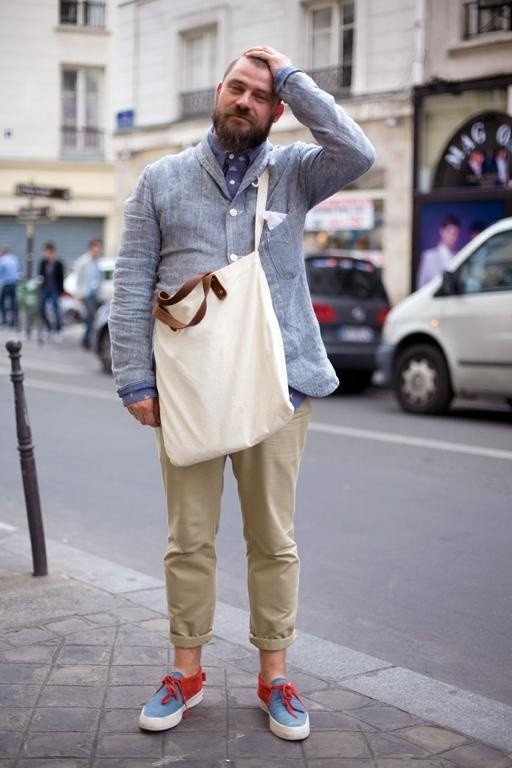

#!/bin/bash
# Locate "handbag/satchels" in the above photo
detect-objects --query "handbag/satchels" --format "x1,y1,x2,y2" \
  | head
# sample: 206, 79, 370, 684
150, 255, 298, 468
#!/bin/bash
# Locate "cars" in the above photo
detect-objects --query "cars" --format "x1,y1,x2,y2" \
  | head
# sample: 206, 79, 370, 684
55, 256, 116, 326
88, 250, 391, 397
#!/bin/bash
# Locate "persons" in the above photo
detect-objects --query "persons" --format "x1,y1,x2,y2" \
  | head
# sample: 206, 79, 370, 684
418, 214, 462, 287
18, 271, 46, 348
71, 238, 106, 349
37, 242, 68, 343
460, 145, 512, 188
103, 41, 382, 743
460, 220, 493, 291
2, 246, 24, 329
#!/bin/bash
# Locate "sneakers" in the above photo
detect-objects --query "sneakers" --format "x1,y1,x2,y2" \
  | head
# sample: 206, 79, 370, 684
138, 664, 206, 731
26, 330, 65, 345
256, 670, 312, 740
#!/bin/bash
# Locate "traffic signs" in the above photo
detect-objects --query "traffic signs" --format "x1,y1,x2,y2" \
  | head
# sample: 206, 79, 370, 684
15, 183, 71, 201
16, 206, 56, 225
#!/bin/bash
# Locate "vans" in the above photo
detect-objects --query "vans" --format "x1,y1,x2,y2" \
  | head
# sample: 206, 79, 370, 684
375, 217, 511, 417
